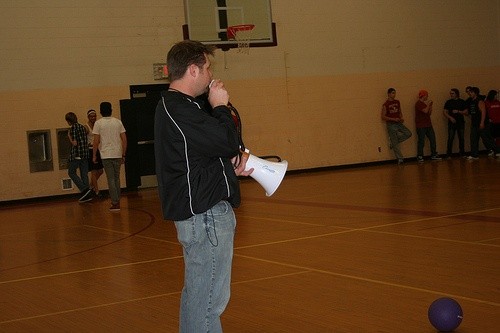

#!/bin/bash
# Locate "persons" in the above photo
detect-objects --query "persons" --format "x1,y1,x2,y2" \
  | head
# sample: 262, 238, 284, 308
413, 89, 443, 164
444, 89, 468, 161
466, 86, 500, 160
153, 40, 254, 333
380, 87, 412, 167
92, 102, 127, 212
64, 112, 93, 203
84, 109, 107, 200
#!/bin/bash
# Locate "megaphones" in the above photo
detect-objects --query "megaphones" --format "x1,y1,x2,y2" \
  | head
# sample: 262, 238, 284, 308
230, 146, 288, 197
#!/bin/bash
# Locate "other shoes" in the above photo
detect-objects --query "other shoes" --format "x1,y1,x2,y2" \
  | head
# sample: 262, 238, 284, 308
89, 188, 93, 191
95, 193, 102, 198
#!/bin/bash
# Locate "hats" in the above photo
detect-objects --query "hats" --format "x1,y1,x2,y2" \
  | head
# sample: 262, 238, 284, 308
419, 90, 428, 97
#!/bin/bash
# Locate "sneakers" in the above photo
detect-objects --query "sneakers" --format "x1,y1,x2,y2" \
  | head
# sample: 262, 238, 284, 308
399, 159, 403, 164
80, 196, 92, 202
431, 154, 443, 159
79, 187, 91, 200
417, 155, 424, 161
388, 136, 394, 149
446, 150, 500, 161
109, 201, 121, 210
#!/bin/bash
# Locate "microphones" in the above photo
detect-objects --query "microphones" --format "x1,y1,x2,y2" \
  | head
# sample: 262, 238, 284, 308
209, 80, 215, 88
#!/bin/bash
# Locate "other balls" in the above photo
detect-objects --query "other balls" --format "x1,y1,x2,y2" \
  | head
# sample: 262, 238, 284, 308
428, 297, 464, 333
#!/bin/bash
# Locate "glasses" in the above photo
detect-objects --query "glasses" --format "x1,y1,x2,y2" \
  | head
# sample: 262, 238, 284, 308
450, 93, 455, 94
